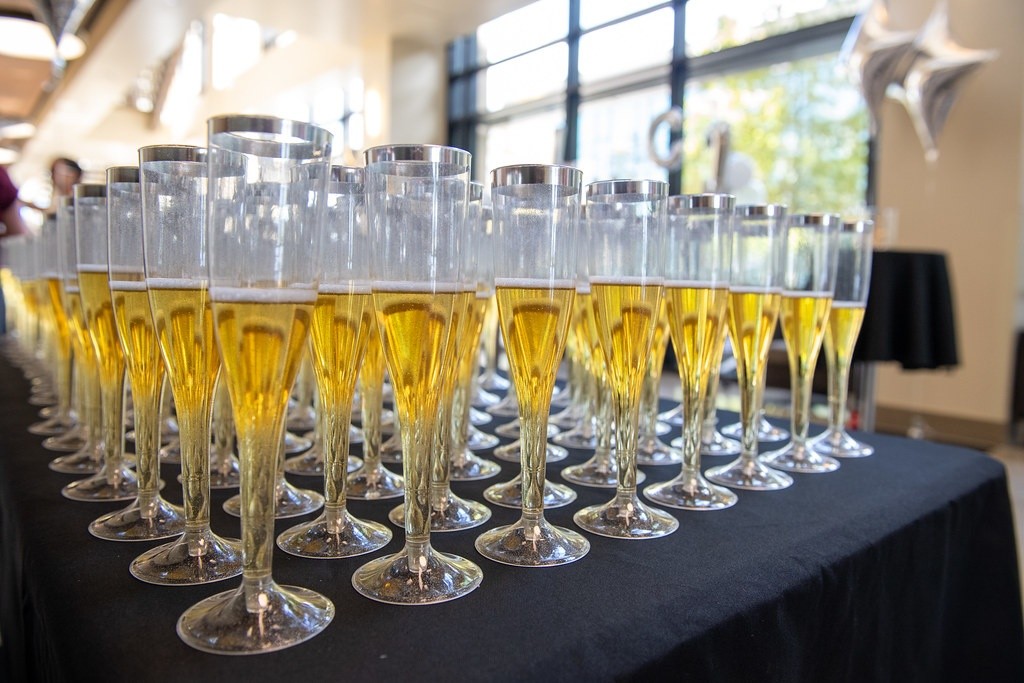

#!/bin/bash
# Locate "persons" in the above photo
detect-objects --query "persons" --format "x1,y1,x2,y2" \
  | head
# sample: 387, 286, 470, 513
36, 158, 82, 217
0, 166, 36, 336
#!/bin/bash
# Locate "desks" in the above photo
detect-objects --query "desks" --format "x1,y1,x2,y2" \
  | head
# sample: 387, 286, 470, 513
0, 366, 1024, 683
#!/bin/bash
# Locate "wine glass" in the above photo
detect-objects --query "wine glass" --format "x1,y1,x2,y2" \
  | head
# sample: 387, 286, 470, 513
0, 110, 877, 658
